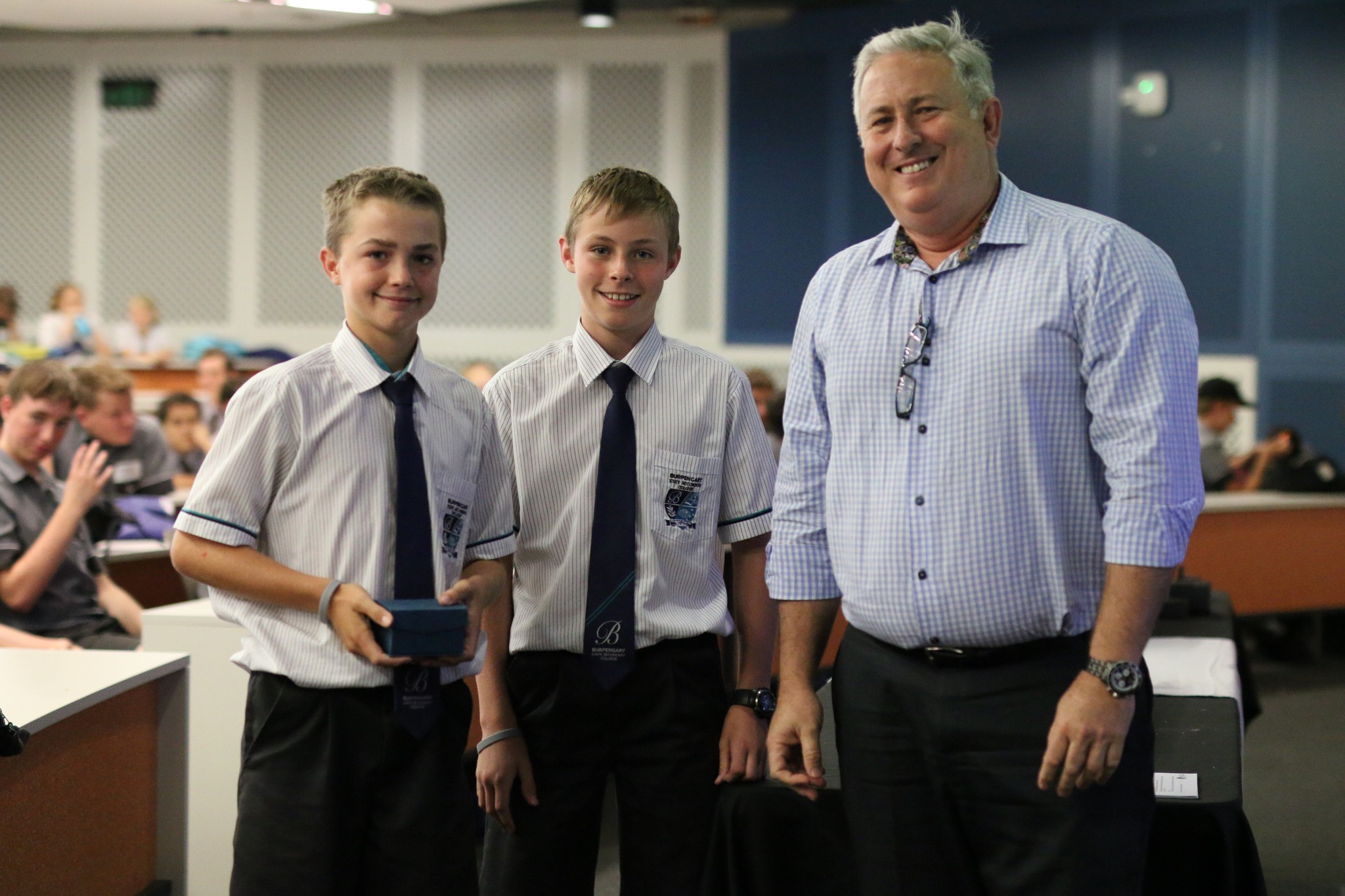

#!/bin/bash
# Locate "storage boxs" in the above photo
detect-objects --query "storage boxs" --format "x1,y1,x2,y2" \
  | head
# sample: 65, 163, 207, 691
369, 598, 471, 659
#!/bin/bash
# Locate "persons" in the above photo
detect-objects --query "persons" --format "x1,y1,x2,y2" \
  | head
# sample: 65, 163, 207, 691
475, 167, 777, 896
759, 10, 1204, 896
0, 283, 238, 655
742, 361, 786, 469
461, 357, 498, 395
171, 167, 519, 896
1192, 375, 1293, 492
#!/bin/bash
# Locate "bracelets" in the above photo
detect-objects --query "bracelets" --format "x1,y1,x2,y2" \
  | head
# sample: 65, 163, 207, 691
476, 727, 517, 754
316, 579, 339, 625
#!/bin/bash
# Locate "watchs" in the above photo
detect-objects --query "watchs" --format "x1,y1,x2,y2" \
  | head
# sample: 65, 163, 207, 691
1083, 655, 1144, 699
730, 686, 776, 723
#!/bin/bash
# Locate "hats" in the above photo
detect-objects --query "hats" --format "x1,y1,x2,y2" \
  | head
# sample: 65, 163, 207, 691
1199, 378, 1250, 406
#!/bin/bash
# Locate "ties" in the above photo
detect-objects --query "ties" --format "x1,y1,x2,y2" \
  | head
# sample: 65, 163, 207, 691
581, 362, 637, 687
380, 373, 441, 719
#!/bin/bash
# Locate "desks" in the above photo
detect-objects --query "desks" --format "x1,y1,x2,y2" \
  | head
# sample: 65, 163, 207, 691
67, 355, 270, 397
722, 587, 1271, 896
95, 537, 193, 607
0, 597, 254, 896
1174, 486, 1345, 659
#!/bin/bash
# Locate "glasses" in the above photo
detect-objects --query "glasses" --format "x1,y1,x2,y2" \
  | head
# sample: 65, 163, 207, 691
894, 318, 933, 421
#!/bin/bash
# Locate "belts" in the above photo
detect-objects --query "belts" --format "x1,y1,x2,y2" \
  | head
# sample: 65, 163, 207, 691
924, 641, 1013, 667
